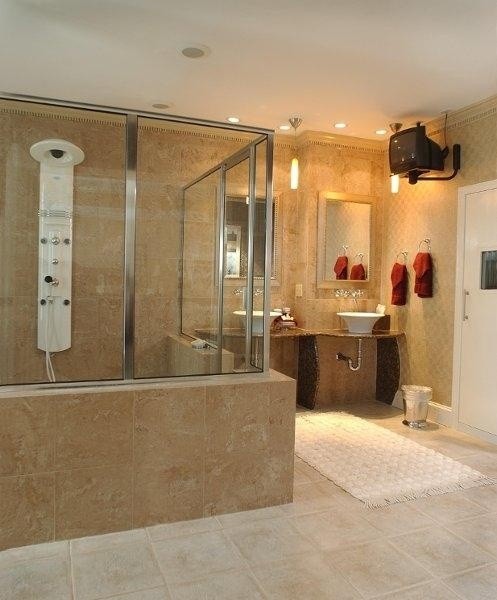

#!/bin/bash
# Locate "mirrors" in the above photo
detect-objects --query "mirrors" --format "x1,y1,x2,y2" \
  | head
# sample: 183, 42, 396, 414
214, 185, 283, 289
317, 191, 379, 289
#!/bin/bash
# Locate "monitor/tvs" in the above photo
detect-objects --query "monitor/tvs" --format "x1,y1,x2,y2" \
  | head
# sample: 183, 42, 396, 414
388, 126, 444, 179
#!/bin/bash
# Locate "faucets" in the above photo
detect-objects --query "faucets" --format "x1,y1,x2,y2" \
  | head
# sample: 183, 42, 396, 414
254, 288, 263, 298
351, 290, 364, 301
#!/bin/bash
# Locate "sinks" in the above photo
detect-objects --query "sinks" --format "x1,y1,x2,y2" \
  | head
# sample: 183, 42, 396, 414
233, 311, 282, 335
336, 312, 385, 334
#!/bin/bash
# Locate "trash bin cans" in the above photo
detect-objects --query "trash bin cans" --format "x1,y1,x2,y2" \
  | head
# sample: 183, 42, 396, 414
402, 384, 433, 428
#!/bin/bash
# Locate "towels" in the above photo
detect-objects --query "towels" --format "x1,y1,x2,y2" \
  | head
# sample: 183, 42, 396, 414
413, 252, 434, 299
334, 256, 366, 281
390, 263, 408, 306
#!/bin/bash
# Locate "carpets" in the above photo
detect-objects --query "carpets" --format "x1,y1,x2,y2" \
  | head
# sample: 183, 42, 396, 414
293, 407, 497, 512
